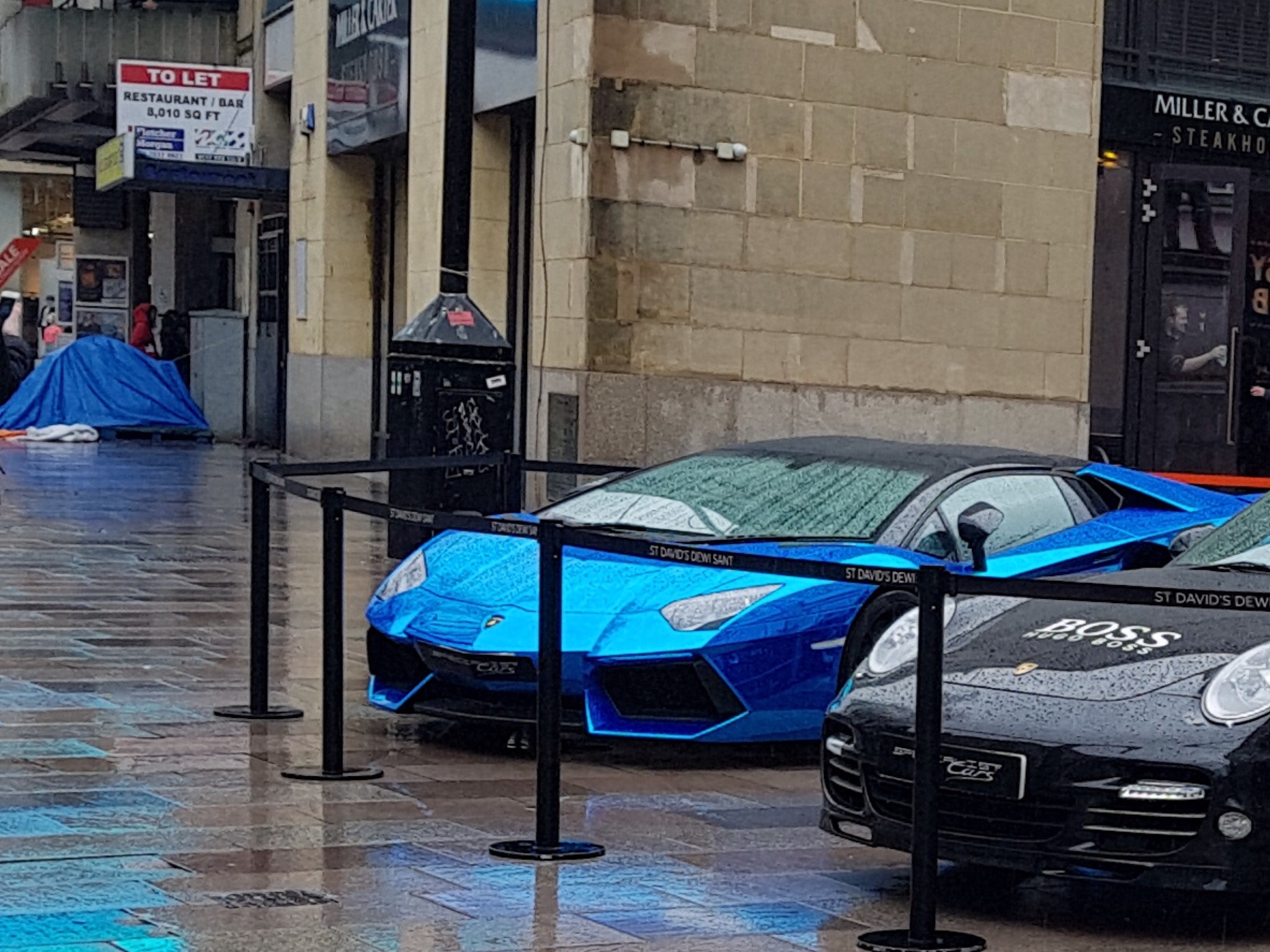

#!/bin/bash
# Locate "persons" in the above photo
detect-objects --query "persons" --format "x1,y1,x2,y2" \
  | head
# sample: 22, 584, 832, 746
159, 310, 189, 371
131, 303, 158, 359
37, 295, 55, 341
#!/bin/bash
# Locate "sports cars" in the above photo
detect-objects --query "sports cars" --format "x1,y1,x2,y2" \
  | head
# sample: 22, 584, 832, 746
818, 486, 1269, 896
363, 432, 1270, 742
